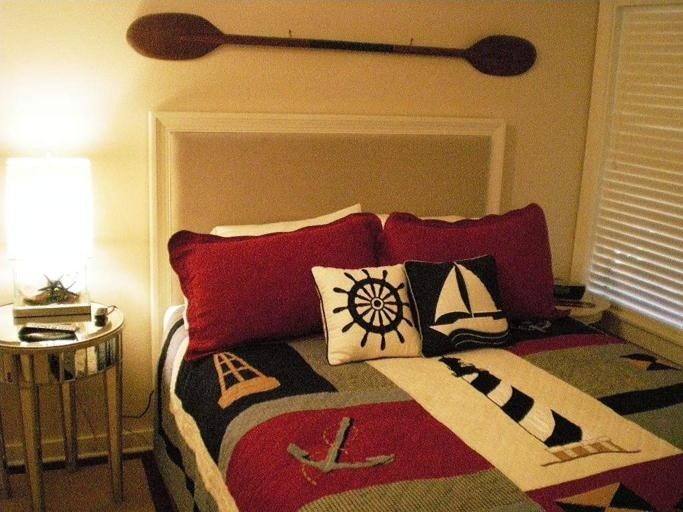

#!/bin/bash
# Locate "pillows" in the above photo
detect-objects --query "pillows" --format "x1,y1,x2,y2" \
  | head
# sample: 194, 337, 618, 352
166, 210, 382, 366
381, 203, 559, 337
401, 254, 520, 360
310, 263, 422, 366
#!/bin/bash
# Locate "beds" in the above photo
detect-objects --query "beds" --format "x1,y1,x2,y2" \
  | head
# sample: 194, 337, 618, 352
150, 110, 683, 512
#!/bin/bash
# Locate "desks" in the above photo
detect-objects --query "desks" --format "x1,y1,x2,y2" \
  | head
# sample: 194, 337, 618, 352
0, 302, 125, 511
557, 295, 612, 326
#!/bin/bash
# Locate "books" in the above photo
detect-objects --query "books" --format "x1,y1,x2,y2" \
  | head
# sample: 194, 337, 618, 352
12, 296, 90, 318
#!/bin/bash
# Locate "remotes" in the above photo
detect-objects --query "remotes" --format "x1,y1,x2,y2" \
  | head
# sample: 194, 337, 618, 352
94, 308, 106, 320
23, 323, 78, 332
